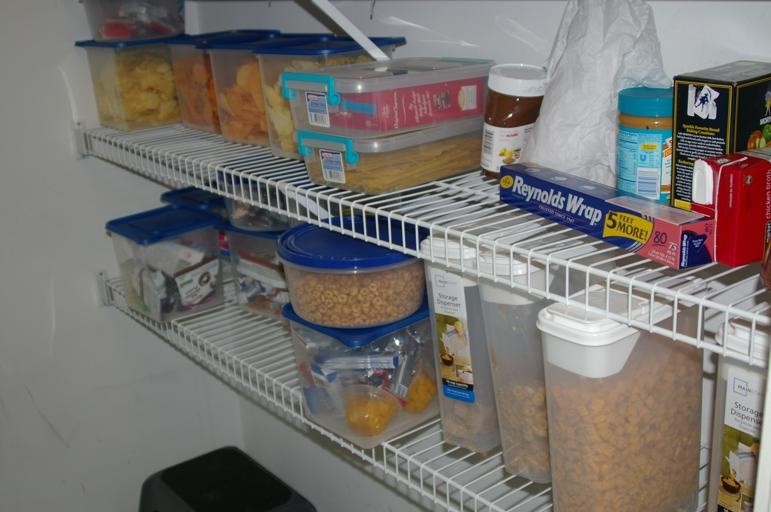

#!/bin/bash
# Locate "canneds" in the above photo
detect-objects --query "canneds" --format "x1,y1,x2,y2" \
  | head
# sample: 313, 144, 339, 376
614, 86, 674, 204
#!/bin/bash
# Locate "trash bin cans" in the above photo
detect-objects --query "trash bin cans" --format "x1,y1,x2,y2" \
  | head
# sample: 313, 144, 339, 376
139, 446, 317, 512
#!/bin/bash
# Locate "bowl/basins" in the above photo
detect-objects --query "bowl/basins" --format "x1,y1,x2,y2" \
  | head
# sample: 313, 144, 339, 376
273, 214, 426, 330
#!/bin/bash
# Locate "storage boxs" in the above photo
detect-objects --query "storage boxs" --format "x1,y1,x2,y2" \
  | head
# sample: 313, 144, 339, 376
71, 2, 498, 449
